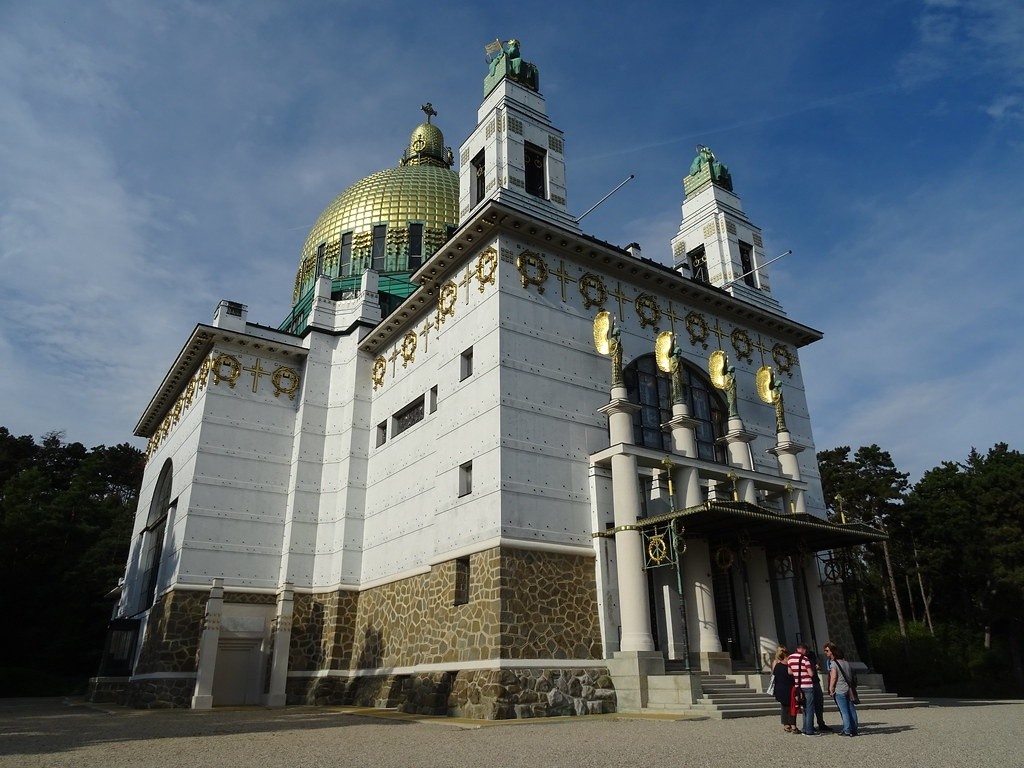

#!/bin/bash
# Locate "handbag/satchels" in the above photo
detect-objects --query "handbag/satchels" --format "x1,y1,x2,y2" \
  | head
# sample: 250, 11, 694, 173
766, 674, 775, 696
849, 686, 860, 704
795, 687, 804, 702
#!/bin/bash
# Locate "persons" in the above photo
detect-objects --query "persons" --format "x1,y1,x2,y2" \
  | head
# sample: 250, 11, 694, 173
770, 643, 859, 737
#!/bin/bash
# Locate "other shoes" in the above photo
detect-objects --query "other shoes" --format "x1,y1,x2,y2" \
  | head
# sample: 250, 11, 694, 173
802, 731, 822, 737
784, 725, 802, 734
819, 725, 833, 732
838, 730, 858, 736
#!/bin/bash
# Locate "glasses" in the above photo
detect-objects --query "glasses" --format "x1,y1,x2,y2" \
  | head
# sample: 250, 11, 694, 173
824, 650, 829, 652
